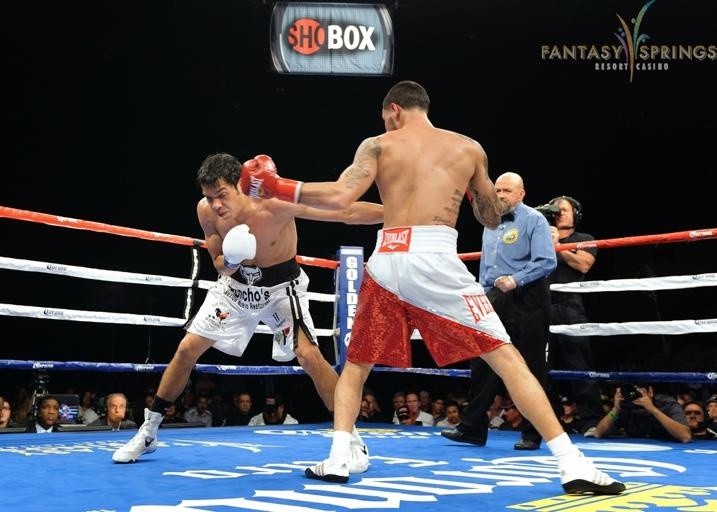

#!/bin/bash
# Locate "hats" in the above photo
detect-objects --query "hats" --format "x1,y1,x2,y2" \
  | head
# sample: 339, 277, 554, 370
261, 393, 286, 413
396, 405, 409, 417
561, 397, 573, 406
501, 400, 514, 409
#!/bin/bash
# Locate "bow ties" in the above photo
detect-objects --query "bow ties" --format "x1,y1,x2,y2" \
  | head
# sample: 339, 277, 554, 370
501, 212, 514, 224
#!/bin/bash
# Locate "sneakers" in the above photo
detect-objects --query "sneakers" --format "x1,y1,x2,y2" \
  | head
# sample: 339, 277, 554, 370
584, 427, 597, 437
560, 464, 625, 494
305, 424, 369, 483
112, 408, 166, 462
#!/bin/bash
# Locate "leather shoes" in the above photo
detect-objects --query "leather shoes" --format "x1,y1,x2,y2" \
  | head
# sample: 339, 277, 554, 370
441, 429, 486, 445
514, 440, 539, 450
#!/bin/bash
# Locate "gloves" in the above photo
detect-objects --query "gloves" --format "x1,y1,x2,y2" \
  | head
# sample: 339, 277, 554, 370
222, 224, 257, 270
236, 154, 303, 204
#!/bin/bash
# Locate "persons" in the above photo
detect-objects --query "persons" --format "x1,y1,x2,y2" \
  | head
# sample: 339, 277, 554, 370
439, 168, 559, 451
111, 150, 385, 475
537, 195, 605, 439
2, 367, 716, 444
239, 78, 627, 495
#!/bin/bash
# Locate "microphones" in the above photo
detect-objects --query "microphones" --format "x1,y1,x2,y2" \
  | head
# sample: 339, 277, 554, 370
559, 225, 576, 231
121, 417, 128, 421
35, 415, 42, 423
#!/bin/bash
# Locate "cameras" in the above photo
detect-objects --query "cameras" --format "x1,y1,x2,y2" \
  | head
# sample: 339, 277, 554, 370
619, 382, 643, 409
534, 204, 561, 226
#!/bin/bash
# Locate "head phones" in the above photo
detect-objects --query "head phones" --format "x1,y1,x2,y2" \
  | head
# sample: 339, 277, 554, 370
31, 396, 62, 417
95, 396, 132, 421
549, 196, 582, 225
683, 401, 712, 426
